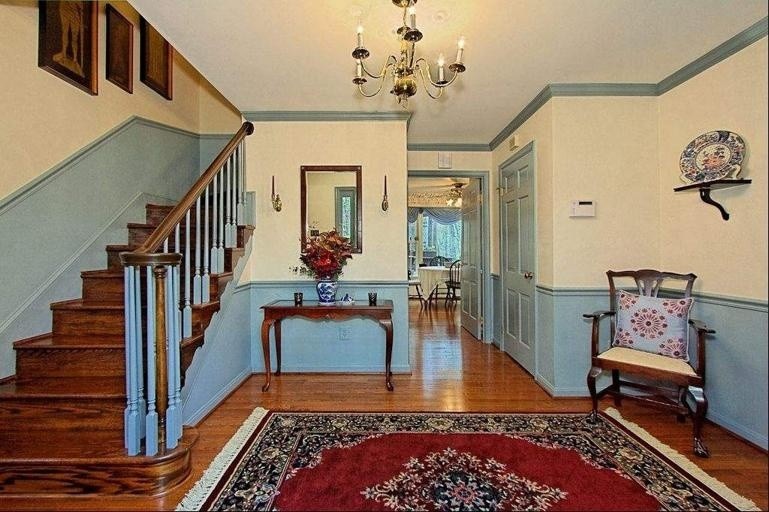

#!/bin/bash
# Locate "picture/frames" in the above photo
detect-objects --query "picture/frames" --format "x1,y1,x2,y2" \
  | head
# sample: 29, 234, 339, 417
104, 3, 135, 94
139, 15, 174, 101
37, 0, 100, 97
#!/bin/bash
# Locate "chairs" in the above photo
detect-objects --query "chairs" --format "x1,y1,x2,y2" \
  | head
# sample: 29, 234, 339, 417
445, 260, 465, 308
428, 255, 449, 306
407, 269, 424, 308
582, 268, 717, 459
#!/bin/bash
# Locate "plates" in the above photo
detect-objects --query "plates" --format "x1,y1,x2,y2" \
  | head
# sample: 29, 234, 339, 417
679, 130, 746, 184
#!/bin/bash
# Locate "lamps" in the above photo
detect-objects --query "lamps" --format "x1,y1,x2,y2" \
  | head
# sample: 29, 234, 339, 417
350, 0, 465, 113
445, 184, 463, 208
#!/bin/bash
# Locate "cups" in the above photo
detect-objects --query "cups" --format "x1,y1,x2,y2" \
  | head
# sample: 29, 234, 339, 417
368, 293, 377, 303
294, 293, 303, 304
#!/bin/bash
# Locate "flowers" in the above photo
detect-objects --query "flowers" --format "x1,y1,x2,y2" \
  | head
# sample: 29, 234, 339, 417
289, 226, 353, 280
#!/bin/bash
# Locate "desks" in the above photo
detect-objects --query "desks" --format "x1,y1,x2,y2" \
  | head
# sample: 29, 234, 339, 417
256, 298, 396, 393
417, 266, 461, 311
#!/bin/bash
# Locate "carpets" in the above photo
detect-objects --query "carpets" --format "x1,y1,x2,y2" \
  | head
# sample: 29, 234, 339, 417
173, 406, 765, 512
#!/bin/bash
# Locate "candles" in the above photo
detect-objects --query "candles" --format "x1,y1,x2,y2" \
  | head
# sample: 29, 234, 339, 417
272, 176, 274, 197
384, 176, 386, 195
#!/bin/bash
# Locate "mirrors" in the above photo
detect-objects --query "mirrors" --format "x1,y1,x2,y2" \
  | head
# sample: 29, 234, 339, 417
299, 164, 363, 254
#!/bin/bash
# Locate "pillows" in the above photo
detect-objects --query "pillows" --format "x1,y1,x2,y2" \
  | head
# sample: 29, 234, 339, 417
611, 289, 695, 363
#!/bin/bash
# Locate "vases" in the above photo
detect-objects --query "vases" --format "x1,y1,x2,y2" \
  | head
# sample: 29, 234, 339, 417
316, 276, 339, 305
